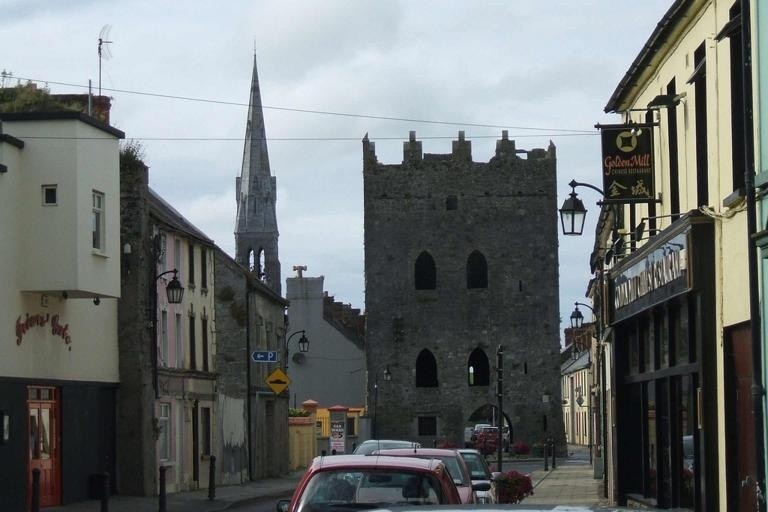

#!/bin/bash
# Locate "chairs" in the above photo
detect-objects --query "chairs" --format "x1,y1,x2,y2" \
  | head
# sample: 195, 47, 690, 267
324, 477, 355, 505
396, 477, 436, 505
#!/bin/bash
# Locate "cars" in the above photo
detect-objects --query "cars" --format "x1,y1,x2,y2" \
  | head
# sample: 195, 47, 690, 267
351, 437, 420, 455
667, 432, 693, 470
275, 454, 462, 512
469, 424, 509, 445
451, 447, 503, 508
351, 447, 491, 507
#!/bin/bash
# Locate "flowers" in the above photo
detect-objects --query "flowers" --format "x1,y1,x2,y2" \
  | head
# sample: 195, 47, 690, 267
493, 470, 534, 502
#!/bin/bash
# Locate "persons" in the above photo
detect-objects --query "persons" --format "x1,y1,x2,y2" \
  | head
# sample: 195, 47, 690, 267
351, 442, 356, 452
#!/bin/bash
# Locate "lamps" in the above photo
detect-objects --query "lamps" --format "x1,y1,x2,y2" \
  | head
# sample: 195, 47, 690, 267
605, 214, 687, 265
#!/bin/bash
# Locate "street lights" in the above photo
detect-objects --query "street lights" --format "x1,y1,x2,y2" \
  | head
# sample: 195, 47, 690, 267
371, 363, 393, 439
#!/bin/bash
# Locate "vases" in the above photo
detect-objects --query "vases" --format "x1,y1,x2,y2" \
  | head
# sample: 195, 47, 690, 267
498, 488, 515, 504
